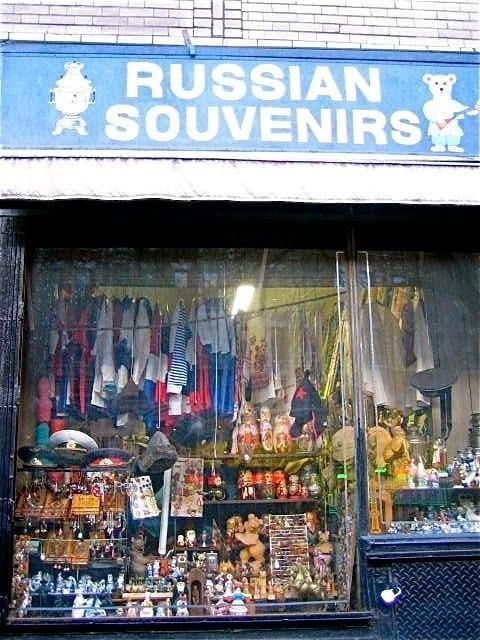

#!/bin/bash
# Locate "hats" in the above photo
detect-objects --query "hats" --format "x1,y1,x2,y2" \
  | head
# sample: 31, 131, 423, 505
142, 428, 177, 475
289, 376, 323, 418
331, 426, 355, 462
17, 445, 61, 469
47, 429, 99, 466
409, 365, 459, 399
82, 447, 132, 470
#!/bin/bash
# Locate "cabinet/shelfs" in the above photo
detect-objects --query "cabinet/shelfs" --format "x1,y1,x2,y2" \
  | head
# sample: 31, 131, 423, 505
13, 467, 128, 609
175, 453, 349, 614
377, 489, 480, 533
